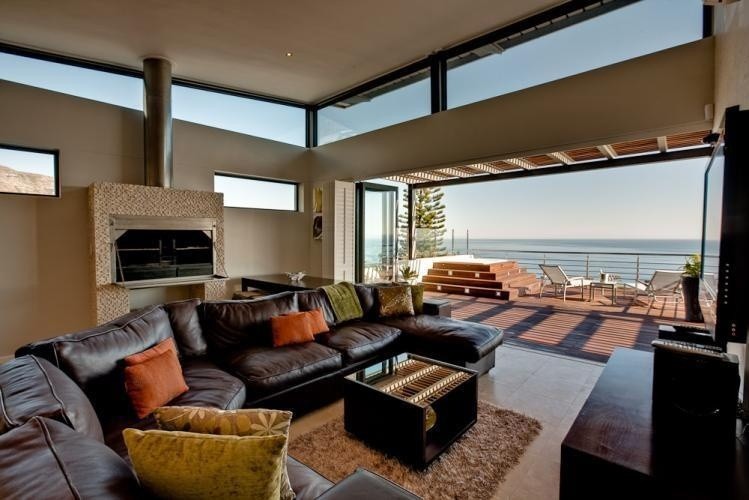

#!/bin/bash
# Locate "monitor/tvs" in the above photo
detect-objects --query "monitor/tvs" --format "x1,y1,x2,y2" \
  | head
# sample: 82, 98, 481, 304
697, 105, 748, 346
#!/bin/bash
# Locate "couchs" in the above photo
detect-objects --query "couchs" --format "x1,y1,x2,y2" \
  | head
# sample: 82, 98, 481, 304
0, 281, 505, 499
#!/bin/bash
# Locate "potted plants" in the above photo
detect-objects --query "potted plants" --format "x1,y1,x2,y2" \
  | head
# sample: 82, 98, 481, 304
677, 253, 705, 323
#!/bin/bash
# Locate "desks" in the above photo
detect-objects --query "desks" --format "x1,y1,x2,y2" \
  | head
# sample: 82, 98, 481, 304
241, 273, 334, 294
559, 347, 745, 499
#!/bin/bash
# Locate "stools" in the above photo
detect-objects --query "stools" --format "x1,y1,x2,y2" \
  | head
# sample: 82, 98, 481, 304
231, 288, 265, 299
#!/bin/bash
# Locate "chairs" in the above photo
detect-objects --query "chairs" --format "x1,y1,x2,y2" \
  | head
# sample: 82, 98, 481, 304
623, 269, 687, 319
538, 263, 595, 302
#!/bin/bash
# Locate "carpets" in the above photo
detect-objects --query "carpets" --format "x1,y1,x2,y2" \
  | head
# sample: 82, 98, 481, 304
287, 398, 543, 500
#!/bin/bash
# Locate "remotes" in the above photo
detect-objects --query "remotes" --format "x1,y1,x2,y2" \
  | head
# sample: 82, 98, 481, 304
651, 340, 726, 360
672, 324, 710, 334
655, 338, 723, 352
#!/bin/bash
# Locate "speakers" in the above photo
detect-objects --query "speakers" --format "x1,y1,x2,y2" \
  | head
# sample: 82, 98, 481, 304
705, 104, 714, 121
652, 353, 739, 454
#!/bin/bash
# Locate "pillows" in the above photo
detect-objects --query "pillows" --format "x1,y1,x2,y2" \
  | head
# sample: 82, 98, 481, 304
377, 281, 425, 318
126, 338, 190, 419
271, 309, 331, 346
123, 405, 296, 500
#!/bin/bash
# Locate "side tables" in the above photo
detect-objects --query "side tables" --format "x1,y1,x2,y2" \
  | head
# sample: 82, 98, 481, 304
589, 281, 617, 305
